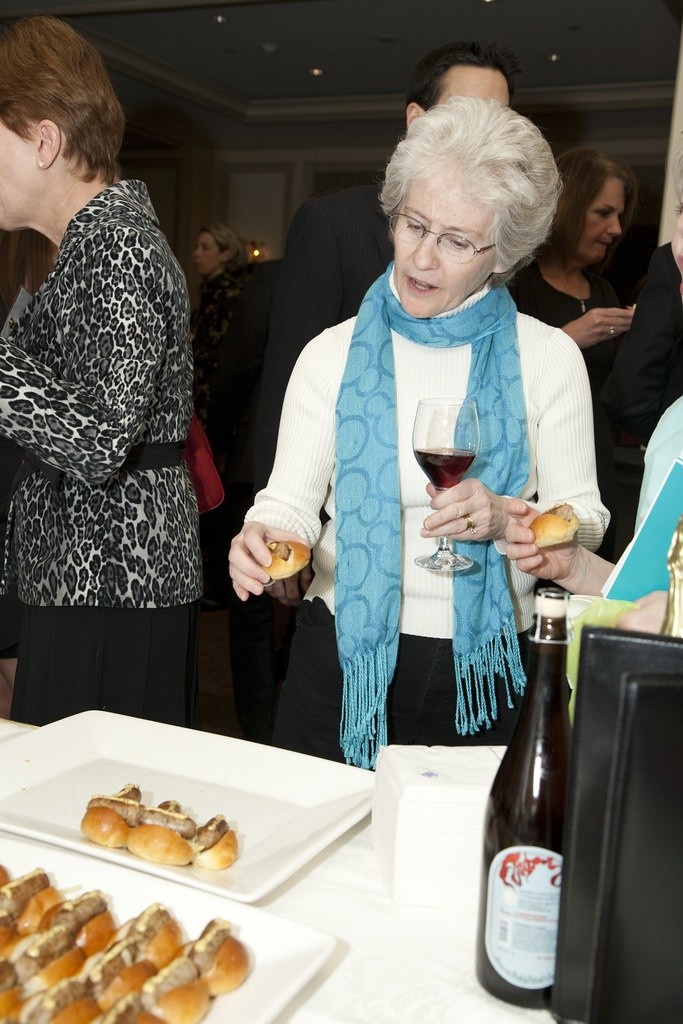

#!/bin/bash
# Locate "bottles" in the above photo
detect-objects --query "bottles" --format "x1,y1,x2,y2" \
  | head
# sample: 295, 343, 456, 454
476, 587, 575, 1009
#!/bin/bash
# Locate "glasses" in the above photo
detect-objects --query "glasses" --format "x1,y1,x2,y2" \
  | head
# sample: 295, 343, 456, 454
386, 213, 497, 264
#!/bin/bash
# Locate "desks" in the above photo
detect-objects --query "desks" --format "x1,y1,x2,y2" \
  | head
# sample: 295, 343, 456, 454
0, 717, 556, 1023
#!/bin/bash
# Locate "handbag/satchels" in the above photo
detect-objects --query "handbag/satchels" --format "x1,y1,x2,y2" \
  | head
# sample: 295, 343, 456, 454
183, 410, 224, 514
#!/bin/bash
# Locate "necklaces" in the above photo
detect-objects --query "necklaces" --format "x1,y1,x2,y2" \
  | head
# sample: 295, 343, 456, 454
552, 263, 588, 312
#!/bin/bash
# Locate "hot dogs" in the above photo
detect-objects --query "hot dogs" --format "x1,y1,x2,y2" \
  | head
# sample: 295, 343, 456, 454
81, 784, 239, 869
262, 542, 310, 579
0, 865, 252, 1024
529, 503, 580, 548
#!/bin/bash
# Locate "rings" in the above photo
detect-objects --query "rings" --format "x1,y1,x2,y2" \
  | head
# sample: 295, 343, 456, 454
464, 516, 474, 529
610, 326, 615, 334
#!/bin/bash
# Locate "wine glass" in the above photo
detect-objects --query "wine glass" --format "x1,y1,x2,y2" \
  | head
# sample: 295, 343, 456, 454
411, 398, 480, 570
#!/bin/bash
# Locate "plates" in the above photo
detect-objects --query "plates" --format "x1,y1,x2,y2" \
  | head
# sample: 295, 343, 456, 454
0, 711, 377, 901
1, 833, 336, 1024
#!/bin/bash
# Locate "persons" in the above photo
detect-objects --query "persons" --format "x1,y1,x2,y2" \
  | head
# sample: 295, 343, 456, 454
507, 145, 683, 636
190, 221, 250, 609
0, 12, 203, 722
237, 38, 522, 747
227, 94, 609, 770
